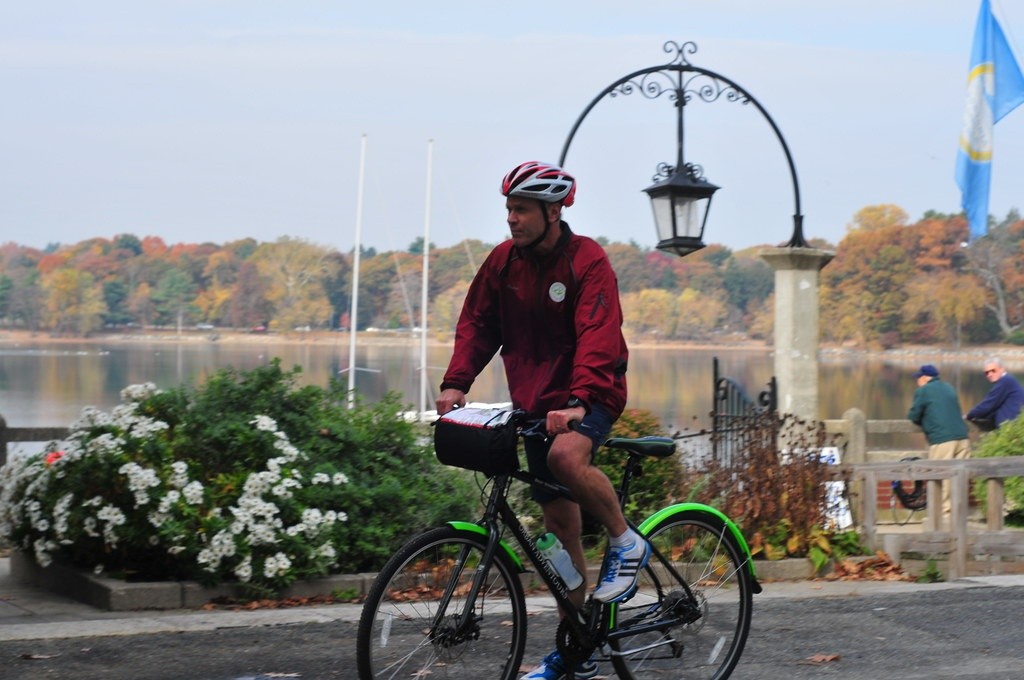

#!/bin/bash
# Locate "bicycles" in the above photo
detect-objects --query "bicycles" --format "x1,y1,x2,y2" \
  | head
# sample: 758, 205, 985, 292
357, 407, 762, 680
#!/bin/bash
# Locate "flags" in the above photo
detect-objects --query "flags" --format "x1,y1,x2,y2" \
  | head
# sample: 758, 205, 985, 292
955, 0, 1024, 250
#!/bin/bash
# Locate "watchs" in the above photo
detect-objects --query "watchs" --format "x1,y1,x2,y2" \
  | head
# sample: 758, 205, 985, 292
568, 395, 589, 411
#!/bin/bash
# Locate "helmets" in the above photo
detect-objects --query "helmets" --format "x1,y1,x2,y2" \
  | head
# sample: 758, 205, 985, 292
501, 161, 576, 207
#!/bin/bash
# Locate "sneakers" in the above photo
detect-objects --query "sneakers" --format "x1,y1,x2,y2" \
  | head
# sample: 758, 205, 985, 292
591, 533, 652, 604
520, 649, 599, 680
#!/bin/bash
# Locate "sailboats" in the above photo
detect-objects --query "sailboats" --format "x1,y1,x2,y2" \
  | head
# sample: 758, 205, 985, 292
384, 138, 514, 446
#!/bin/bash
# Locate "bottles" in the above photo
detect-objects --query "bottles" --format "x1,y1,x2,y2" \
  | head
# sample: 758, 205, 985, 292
535, 532, 584, 591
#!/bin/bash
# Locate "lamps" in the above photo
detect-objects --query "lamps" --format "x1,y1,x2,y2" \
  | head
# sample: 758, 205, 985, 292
640, 162, 722, 260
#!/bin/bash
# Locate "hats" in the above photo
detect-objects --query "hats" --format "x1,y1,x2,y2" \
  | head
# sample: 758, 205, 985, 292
914, 364, 938, 377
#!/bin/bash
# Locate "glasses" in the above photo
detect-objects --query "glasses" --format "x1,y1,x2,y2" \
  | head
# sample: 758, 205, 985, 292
985, 369, 998, 376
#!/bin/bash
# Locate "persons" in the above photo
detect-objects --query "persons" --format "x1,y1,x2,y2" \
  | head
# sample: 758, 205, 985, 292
434, 161, 652, 680
907, 364, 972, 518
962, 357, 1024, 428
